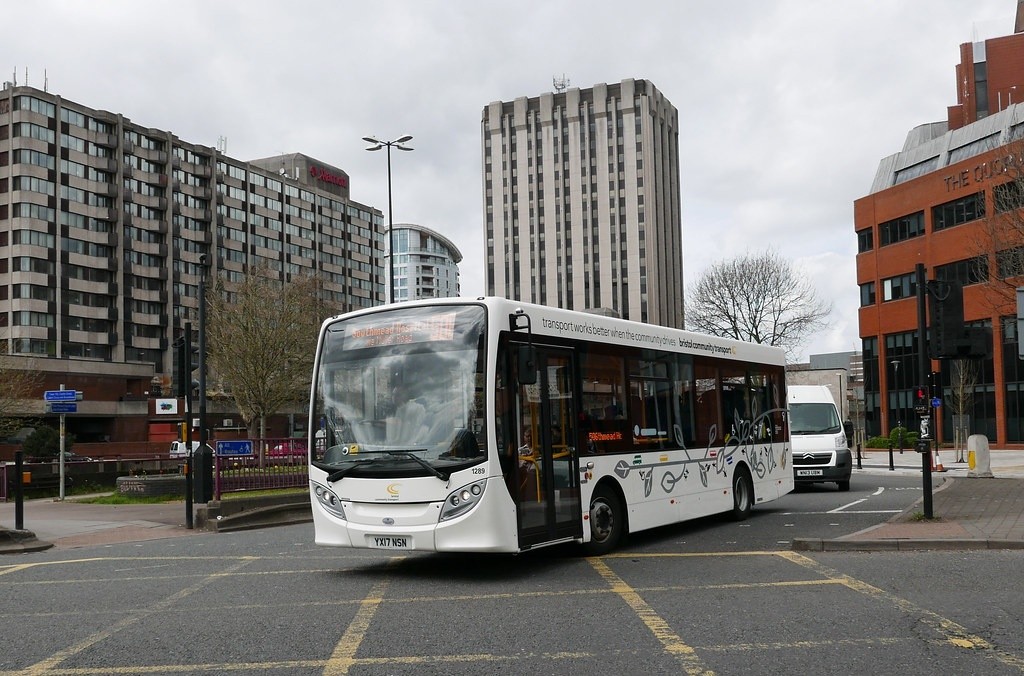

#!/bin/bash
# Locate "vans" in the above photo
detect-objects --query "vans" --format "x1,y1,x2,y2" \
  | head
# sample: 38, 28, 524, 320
170, 441, 215, 459
783, 385, 853, 492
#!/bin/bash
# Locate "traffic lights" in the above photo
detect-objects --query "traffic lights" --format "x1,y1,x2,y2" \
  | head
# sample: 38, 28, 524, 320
911, 384, 930, 418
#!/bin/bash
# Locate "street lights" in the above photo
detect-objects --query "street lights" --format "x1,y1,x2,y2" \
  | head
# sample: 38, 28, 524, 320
363, 133, 415, 304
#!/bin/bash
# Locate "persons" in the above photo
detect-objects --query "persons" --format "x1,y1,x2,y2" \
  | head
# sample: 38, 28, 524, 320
518, 428, 542, 475
605, 405, 624, 419
378, 384, 410, 427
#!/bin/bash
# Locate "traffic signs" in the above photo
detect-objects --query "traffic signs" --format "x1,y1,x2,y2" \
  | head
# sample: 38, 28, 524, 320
43, 390, 83, 413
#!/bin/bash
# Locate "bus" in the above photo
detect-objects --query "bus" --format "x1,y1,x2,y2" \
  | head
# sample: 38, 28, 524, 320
306, 295, 793, 563
